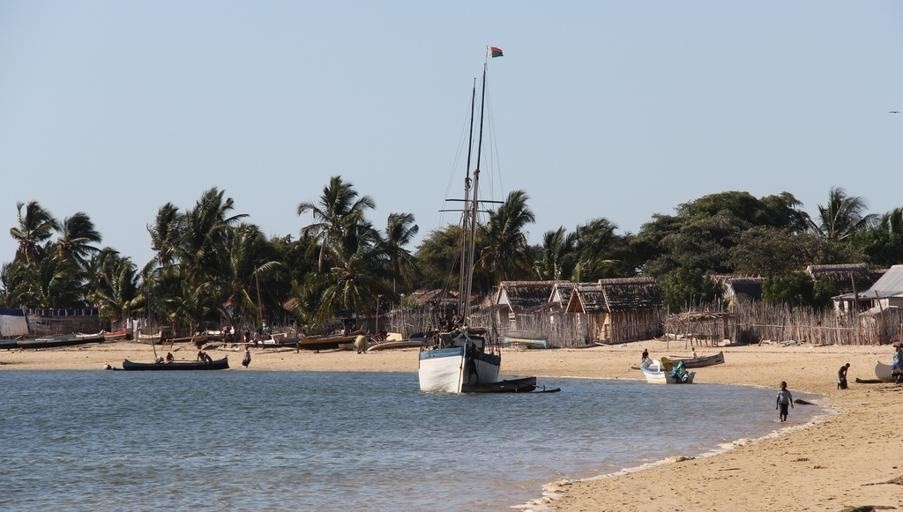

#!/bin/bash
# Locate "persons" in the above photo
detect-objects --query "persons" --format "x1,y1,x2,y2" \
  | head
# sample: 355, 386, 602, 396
224, 325, 264, 348
241, 346, 250, 368
641, 348, 648, 362
352, 334, 366, 353
775, 380, 795, 423
449, 309, 463, 329
165, 352, 174, 363
835, 361, 851, 390
891, 343, 902, 385
463, 343, 481, 383
195, 350, 212, 362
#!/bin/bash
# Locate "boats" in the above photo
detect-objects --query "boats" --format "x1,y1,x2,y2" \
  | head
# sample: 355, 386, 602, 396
874, 360, 901, 382
641, 356, 695, 385
461, 376, 536, 393
660, 350, 724, 371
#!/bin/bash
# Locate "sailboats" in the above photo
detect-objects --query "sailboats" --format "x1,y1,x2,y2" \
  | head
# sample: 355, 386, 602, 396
122, 271, 230, 371
418, 43, 506, 393
